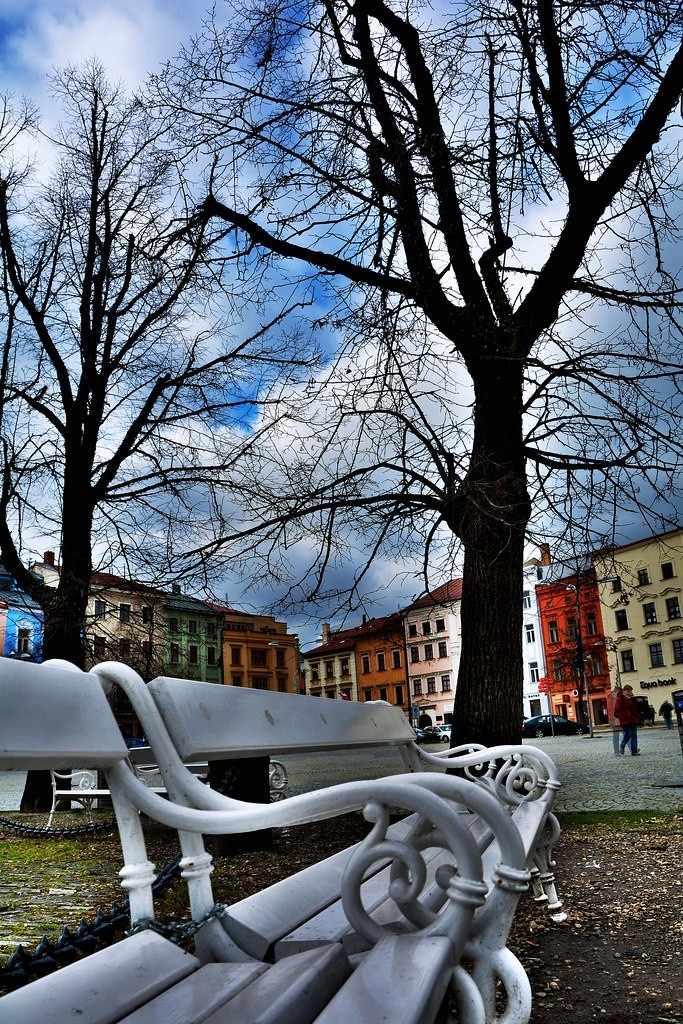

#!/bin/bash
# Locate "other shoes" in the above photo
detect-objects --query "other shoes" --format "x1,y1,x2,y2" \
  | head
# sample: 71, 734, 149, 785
619, 744, 625, 755
632, 751, 641, 756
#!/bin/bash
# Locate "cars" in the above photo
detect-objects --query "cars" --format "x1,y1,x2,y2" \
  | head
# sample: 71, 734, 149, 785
412, 724, 451, 744
522, 715, 590, 738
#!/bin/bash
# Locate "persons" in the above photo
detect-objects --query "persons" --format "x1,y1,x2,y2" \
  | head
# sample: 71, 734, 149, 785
646, 700, 675, 729
614, 685, 642, 756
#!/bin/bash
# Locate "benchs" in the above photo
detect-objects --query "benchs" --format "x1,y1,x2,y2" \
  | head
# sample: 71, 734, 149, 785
0, 657, 563, 1024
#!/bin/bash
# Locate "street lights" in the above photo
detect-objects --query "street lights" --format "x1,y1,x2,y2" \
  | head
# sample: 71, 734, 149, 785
542, 576, 617, 738
268, 636, 324, 694
